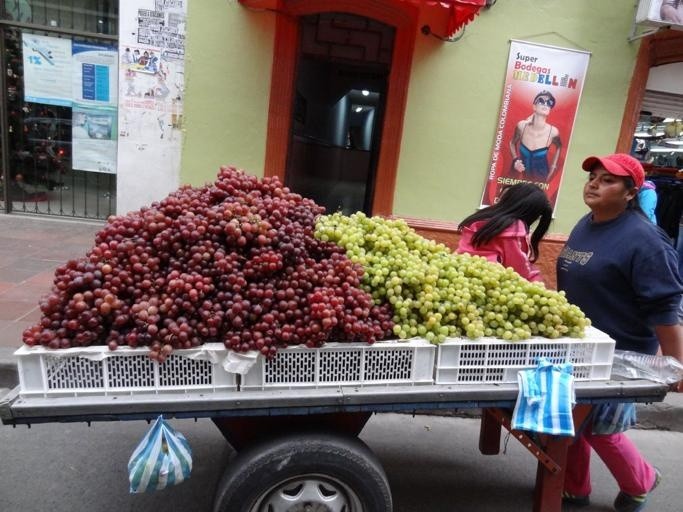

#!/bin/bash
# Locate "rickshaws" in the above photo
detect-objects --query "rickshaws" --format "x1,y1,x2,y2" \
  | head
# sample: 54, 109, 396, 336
0, 348, 683, 511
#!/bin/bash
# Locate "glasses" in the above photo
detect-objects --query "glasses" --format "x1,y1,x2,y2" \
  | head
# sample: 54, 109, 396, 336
535, 97, 554, 107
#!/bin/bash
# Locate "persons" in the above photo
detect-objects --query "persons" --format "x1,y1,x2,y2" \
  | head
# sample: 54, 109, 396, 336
557, 154, 683, 512
454, 182, 552, 291
636, 171, 658, 225
508, 90, 562, 184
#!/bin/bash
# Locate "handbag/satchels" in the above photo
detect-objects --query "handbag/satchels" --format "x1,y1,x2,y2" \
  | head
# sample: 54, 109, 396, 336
127, 412, 195, 495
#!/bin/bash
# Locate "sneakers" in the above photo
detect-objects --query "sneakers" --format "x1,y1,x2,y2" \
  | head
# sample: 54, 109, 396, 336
561, 488, 590, 507
614, 466, 663, 511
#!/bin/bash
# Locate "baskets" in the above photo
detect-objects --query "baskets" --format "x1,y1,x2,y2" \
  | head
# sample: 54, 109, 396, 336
436, 322, 616, 386
12, 318, 616, 406
239, 336, 436, 391
13, 342, 240, 400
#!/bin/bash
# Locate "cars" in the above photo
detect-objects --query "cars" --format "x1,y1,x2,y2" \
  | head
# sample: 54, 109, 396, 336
22, 116, 70, 163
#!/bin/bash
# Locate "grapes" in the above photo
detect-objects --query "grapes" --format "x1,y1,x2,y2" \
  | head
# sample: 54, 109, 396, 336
22, 164, 592, 363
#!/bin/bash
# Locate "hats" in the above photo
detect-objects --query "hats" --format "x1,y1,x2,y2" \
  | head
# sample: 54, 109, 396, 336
581, 153, 645, 190
533, 89, 555, 109
